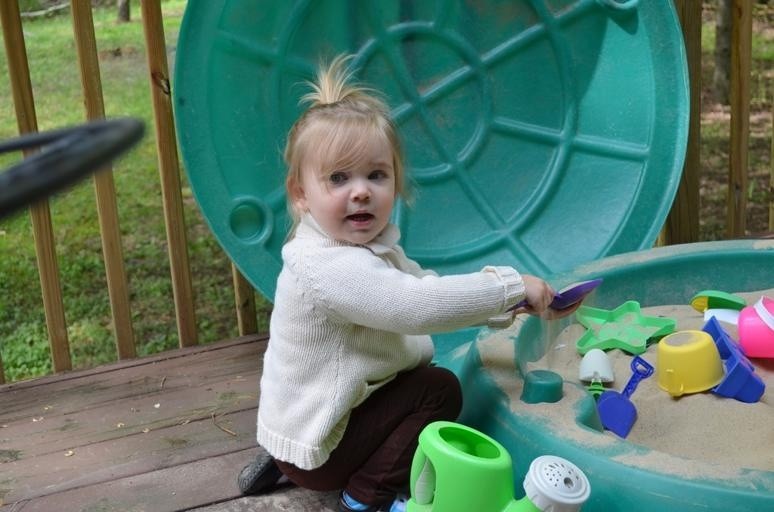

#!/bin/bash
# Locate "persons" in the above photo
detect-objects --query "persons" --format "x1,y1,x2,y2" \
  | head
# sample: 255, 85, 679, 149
234, 44, 560, 511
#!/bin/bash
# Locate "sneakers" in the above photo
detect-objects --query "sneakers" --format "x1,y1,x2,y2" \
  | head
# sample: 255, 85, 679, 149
237, 449, 283, 495
336, 488, 392, 512
